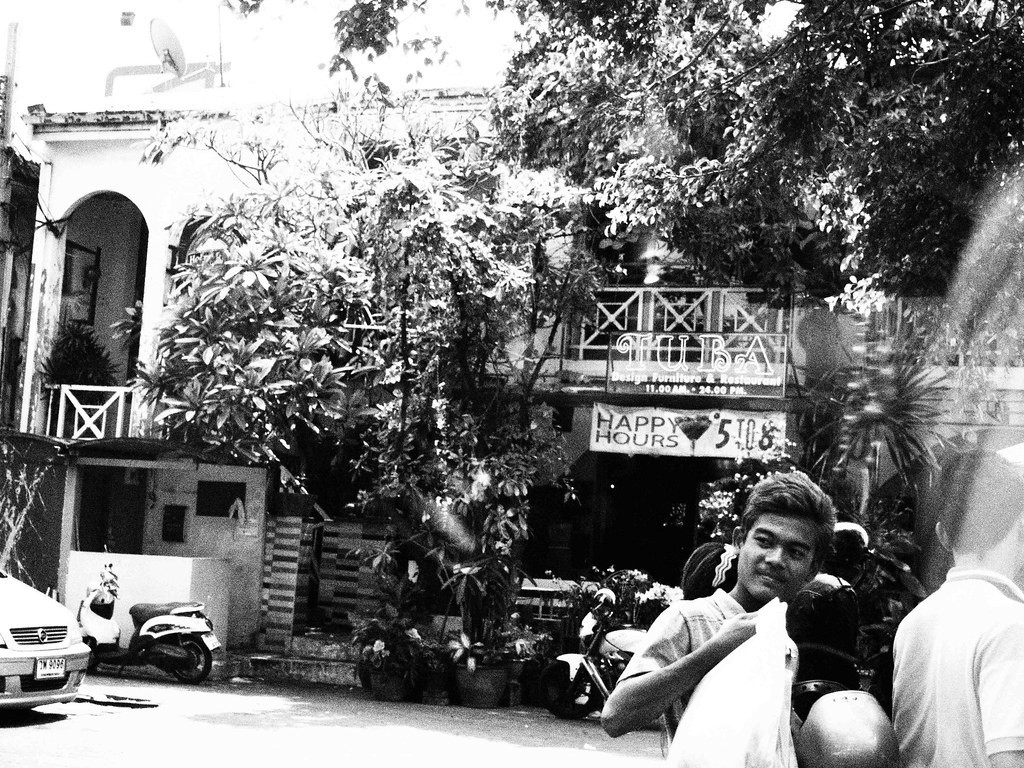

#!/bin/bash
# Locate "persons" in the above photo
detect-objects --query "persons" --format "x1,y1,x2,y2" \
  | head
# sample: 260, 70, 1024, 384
682, 449, 1024, 768
604, 470, 835, 768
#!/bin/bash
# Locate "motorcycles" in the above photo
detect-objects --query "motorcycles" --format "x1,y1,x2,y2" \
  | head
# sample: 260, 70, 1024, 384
541, 575, 650, 721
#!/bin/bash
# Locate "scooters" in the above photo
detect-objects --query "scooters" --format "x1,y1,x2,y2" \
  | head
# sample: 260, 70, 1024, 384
75, 544, 221, 684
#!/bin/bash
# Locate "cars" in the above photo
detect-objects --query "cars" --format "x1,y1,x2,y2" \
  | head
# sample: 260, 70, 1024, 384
0, 568, 93, 708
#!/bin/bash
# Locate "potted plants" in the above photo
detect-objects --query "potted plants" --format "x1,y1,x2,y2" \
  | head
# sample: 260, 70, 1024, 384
348, 549, 429, 702
44, 323, 115, 437
441, 556, 513, 708
